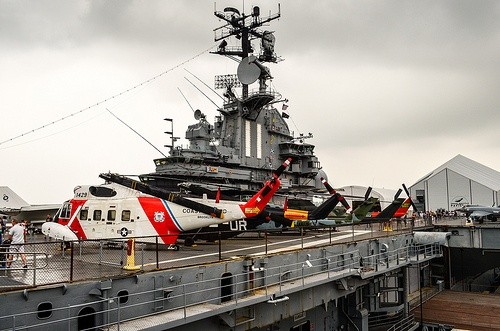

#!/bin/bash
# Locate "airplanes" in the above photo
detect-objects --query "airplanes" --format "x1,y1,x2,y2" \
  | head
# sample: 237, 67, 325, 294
176, 176, 420, 247
41, 158, 292, 252
453, 202, 500, 226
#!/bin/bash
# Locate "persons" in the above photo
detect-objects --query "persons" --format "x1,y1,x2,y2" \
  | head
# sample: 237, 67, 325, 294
404, 208, 457, 227
0, 213, 53, 272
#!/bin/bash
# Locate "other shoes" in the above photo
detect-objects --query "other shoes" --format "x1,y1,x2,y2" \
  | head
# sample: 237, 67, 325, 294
6, 266, 11, 269
22, 264, 28, 268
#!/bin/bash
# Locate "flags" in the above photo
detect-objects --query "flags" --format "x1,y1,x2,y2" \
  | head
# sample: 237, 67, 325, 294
282, 104, 290, 119
